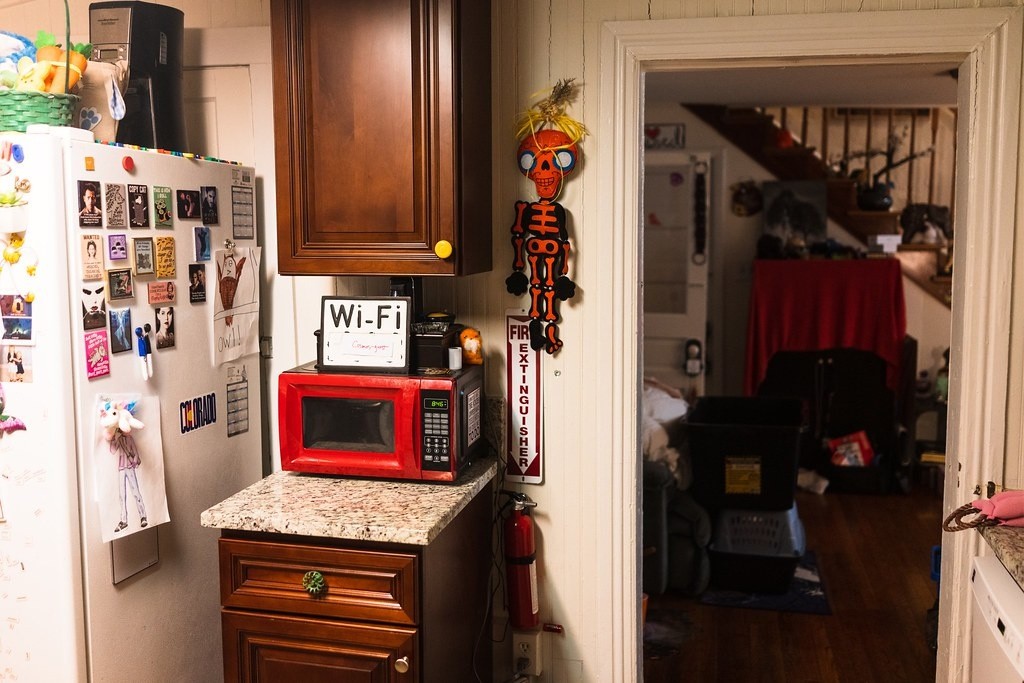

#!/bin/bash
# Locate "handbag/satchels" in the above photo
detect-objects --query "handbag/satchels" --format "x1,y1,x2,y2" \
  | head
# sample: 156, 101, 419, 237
943, 490, 1024, 532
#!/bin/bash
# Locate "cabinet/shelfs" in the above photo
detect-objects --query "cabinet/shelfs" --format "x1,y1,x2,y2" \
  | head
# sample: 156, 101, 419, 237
217, 482, 494, 683
271, 0, 494, 277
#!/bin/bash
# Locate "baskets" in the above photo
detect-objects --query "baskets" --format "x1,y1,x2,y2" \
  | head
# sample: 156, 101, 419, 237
1, 0, 83, 133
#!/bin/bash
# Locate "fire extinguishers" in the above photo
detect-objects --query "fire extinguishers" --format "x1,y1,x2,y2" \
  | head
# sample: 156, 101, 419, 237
495, 488, 542, 630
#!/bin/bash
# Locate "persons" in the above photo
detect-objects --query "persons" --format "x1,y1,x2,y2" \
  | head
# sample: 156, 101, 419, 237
166, 282, 174, 299
190, 267, 204, 292
156, 307, 174, 347
79, 183, 101, 217
87, 240, 98, 262
202, 187, 217, 213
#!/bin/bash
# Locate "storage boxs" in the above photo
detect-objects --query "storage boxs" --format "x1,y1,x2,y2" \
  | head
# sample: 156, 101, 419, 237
711, 499, 806, 557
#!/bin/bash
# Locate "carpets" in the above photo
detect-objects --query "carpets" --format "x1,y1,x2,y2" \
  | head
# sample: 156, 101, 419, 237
692, 550, 835, 618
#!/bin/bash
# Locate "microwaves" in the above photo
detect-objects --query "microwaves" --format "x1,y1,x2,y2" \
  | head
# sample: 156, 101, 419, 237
277, 360, 485, 482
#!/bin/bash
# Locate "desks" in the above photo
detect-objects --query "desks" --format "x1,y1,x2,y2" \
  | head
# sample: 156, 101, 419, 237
743, 254, 906, 398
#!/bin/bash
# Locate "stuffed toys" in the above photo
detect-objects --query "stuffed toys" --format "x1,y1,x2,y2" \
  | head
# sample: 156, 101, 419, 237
99, 402, 146, 440
0, 30, 93, 94
